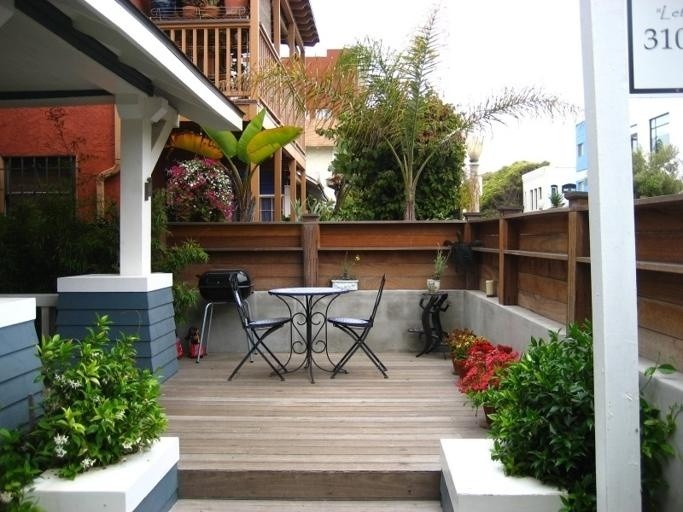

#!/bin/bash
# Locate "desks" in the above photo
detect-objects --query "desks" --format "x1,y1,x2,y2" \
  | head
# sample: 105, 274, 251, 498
407, 292, 454, 359
268, 286, 350, 384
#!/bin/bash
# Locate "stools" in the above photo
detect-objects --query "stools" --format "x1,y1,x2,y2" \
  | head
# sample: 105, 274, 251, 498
196, 300, 258, 363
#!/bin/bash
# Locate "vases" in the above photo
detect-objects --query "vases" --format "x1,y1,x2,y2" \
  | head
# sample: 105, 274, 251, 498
482, 405, 497, 420
224, 0, 248, 17
182, 5, 196, 19
331, 280, 359, 291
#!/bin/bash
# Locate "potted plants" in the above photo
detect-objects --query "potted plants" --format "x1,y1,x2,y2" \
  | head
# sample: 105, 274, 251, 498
203, 0, 219, 17
449, 332, 474, 375
427, 246, 449, 292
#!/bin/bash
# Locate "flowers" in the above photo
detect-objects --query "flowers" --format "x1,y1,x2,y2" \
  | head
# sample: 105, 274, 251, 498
340, 252, 361, 279
456, 337, 523, 400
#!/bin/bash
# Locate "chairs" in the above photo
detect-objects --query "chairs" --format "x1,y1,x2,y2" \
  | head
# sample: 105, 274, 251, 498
228, 272, 293, 381
330, 274, 389, 379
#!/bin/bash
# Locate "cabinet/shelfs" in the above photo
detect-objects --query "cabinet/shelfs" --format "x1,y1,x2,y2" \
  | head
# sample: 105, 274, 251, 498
154, 220, 303, 292
470, 194, 682, 375
318, 221, 470, 292
